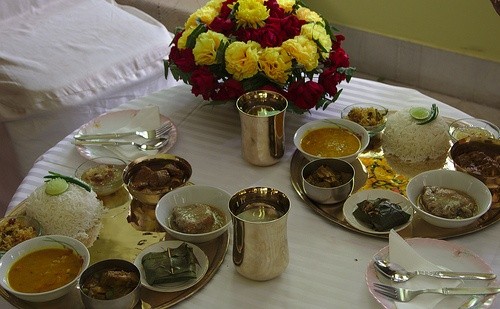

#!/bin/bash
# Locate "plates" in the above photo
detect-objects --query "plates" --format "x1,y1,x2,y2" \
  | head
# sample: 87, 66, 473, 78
343, 190, 412, 235
366, 238, 498, 309
76, 108, 177, 164
133, 240, 209, 293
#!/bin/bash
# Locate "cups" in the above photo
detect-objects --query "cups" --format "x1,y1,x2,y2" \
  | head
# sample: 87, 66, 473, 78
236, 90, 288, 167
228, 187, 291, 282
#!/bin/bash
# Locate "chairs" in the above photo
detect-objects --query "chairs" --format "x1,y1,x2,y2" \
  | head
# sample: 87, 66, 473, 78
0, 0, 183, 176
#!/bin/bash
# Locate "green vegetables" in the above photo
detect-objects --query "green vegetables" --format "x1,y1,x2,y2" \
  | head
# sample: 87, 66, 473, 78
44, 171, 91, 192
416, 104, 439, 125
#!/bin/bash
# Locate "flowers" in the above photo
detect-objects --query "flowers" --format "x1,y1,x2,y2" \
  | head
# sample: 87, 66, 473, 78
164, 0, 357, 113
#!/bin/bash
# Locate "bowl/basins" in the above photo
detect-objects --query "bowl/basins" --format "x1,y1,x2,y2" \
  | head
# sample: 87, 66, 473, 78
155, 185, 233, 244
75, 156, 127, 197
0, 216, 44, 253
341, 104, 388, 138
406, 169, 493, 230
122, 154, 192, 206
79, 259, 142, 309
300, 158, 356, 205
449, 117, 500, 143
0, 235, 90, 303
447, 135, 500, 188
293, 118, 370, 164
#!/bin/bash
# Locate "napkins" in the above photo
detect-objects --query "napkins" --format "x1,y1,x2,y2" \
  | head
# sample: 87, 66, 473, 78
389, 228, 461, 309
102, 105, 161, 160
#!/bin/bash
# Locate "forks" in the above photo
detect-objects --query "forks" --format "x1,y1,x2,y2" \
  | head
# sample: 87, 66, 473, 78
373, 278, 500, 302
74, 123, 172, 139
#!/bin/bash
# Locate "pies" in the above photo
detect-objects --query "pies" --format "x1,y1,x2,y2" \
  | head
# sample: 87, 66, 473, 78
356, 156, 411, 199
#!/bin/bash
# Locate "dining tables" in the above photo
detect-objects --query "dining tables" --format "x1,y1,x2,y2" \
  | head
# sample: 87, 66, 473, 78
3, 76, 500, 309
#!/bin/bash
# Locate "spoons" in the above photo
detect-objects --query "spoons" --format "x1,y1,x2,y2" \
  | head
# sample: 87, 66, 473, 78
74, 136, 168, 152
374, 258, 497, 282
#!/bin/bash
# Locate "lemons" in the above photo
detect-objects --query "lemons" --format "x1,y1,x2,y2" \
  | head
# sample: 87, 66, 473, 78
45, 177, 69, 194
410, 106, 429, 119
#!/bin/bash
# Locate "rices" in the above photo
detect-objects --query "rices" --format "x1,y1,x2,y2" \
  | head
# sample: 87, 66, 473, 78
383, 109, 451, 163
24, 178, 105, 247
